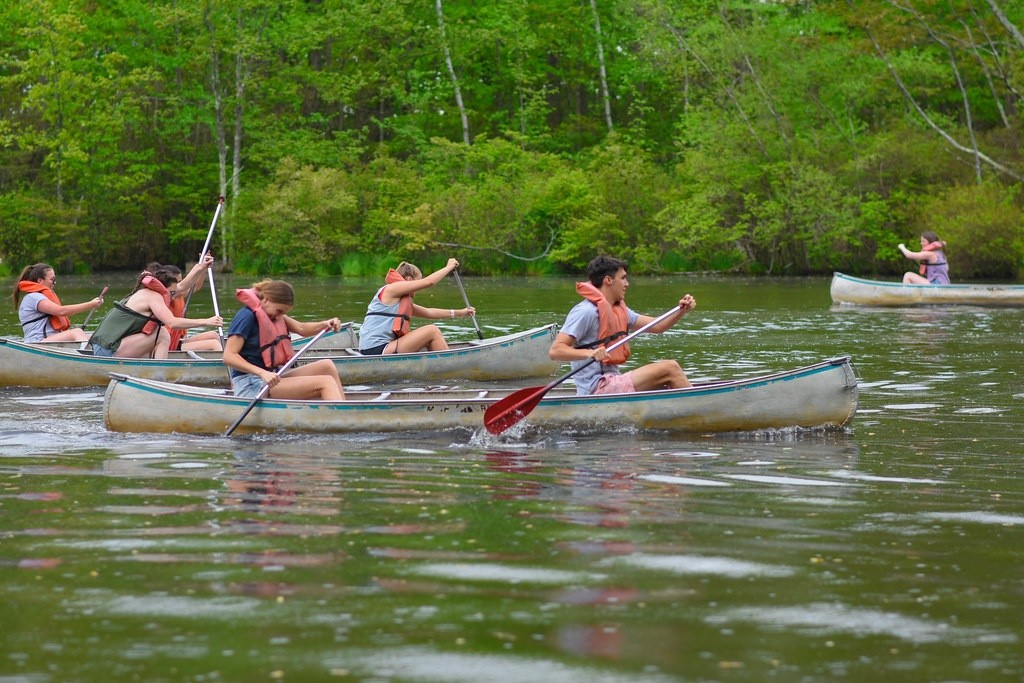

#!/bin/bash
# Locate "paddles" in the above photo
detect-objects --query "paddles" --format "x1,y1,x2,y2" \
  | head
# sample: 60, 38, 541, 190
483, 305, 688, 436
80, 286, 108, 332
222, 324, 332, 436
451, 267, 484, 339
204, 249, 226, 352
182, 194, 226, 317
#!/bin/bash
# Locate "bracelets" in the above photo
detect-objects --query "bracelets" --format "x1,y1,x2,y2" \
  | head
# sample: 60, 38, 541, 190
450, 309, 455, 319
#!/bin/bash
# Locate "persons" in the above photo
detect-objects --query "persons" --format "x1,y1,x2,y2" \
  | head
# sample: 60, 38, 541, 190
358, 257, 476, 356
12, 263, 104, 343
145, 250, 225, 352
548, 256, 697, 396
222, 276, 346, 402
898, 232, 952, 286
89, 269, 224, 362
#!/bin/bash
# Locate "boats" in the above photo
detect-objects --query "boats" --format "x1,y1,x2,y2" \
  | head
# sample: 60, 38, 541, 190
0, 320, 560, 391
290, 325, 359, 352
102, 355, 861, 436
829, 269, 1023, 307
